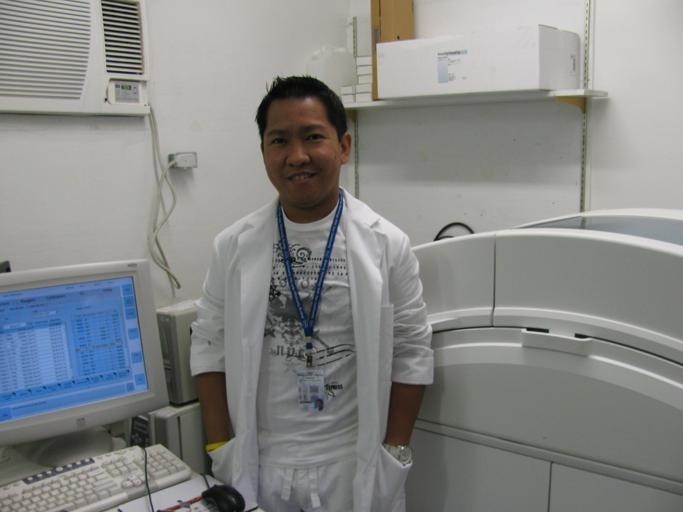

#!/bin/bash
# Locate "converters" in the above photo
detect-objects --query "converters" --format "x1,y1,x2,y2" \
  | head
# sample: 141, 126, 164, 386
174, 153, 196, 168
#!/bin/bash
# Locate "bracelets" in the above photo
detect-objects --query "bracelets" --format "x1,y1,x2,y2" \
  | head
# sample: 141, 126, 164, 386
203, 441, 229, 450
380, 441, 413, 467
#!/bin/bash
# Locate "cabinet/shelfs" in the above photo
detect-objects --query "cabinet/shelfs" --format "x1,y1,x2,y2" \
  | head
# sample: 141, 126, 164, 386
408, 416, 683, 512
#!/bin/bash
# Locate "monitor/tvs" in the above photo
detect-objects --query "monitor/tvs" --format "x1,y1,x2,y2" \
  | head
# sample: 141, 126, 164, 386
0, 259, 170, 468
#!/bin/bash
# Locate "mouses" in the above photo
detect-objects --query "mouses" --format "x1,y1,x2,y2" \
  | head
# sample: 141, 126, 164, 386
202, 484, 245, 512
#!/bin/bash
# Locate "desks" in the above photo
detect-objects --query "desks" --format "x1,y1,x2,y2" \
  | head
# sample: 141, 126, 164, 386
0, 436, 267, 512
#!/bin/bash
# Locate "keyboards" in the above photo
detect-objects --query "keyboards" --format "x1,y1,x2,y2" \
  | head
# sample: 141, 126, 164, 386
0, 443, 192, 512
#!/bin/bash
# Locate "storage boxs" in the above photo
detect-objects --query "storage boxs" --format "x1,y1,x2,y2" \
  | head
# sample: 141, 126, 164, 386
376, 23, 579, 101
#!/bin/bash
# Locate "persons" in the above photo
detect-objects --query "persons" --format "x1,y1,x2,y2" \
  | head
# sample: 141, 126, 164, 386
186, 70, 437, 510
